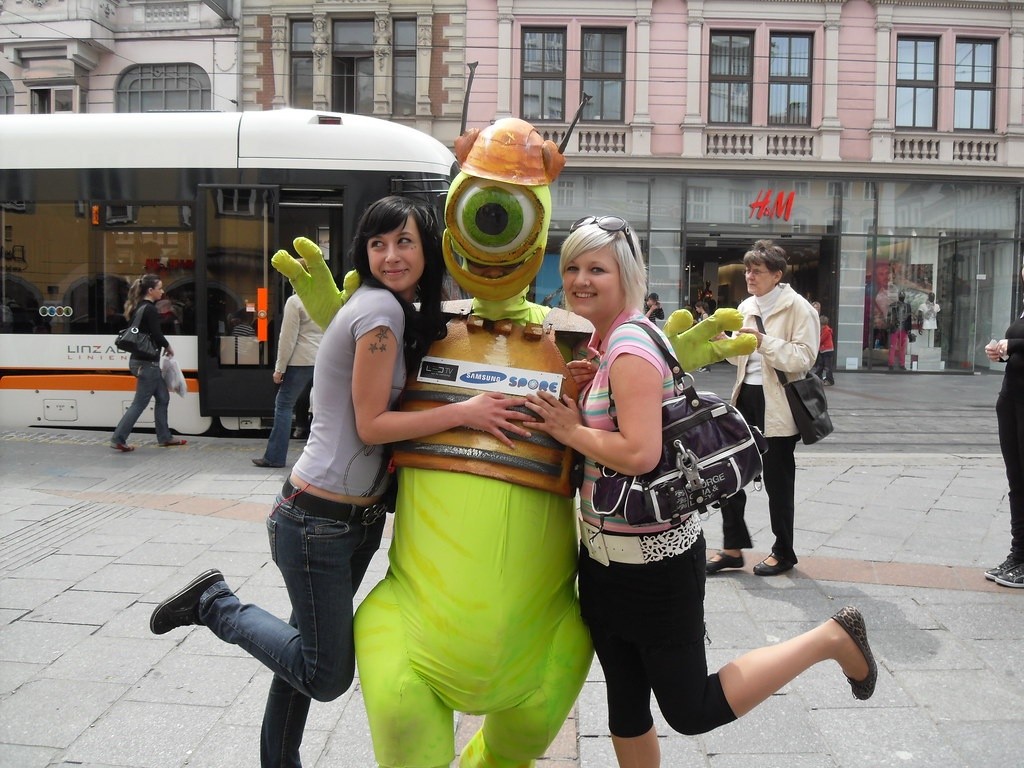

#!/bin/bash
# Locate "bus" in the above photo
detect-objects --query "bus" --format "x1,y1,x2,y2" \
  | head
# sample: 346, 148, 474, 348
0, 109, 462, 435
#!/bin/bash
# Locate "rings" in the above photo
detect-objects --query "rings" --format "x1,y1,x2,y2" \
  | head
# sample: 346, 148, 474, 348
999, 349, 1003, 353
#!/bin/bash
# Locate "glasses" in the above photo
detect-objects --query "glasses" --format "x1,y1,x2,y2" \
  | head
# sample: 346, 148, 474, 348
568, 215, 637, 261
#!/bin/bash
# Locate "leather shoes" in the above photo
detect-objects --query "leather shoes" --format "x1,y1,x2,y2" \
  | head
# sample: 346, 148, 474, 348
705, 550, 744, 572
753, 553, 794, 576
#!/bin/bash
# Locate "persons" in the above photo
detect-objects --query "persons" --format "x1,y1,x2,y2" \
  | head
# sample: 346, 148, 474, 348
918, 292, 941, 335
525, 215, 878, 768
231, 310, 258, 364
644, 292, 665, 327
108, 270, 188, 450
152, 195, 535, 768
985, 255, 1024, 588
811, 300, 836, 387
887, 291, 912, 371
706, 239, 820, 577
865, 256, 933, 349
684, 302, 710, 374
252, 257, 330, 467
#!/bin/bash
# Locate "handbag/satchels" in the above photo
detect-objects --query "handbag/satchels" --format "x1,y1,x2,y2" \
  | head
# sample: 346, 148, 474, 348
115, 304, 160, 361
592, 320, 763, 528
786, 372, 834, 445
162, 352, 187, 397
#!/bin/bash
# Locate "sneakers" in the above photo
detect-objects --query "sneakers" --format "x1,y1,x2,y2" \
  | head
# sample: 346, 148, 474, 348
149, 568, 224, 635
984, 557, 1017, 581
994, 564, 1024, 588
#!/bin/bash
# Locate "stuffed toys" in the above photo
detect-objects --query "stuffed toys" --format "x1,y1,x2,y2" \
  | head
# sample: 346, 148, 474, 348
269, 115, 759, 768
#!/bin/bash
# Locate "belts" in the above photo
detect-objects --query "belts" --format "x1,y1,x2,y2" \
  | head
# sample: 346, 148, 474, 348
281, 475, 398, 527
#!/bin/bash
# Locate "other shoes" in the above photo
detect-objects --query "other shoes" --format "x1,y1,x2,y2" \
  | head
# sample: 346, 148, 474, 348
110, 441, 134, 452
164, 440, 187, 445
822, 377, 835, 386
252, 458, 285, 468
831, 604, 878, 700
